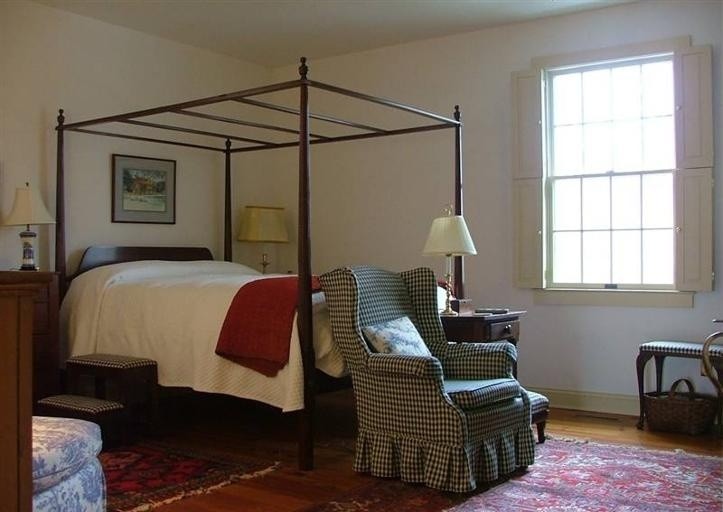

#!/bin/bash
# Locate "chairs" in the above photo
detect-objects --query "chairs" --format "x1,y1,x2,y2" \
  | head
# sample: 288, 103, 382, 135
319, 265, 536, 493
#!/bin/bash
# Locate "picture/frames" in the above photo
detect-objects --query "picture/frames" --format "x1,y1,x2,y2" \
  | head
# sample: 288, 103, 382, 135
111, 153, 177, 224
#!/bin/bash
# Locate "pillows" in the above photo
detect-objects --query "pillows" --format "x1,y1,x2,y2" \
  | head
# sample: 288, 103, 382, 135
362, 317, 431, 358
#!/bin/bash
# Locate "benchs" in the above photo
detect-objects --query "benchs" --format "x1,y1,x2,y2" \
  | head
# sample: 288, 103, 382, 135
636, 341, 723, 433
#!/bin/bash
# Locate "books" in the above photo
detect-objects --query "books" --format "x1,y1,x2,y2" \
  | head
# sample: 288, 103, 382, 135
475, 307, 509, 314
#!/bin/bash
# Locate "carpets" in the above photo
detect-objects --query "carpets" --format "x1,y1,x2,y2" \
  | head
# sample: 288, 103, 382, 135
295, 434, 723, 512
98, 442, 280, 512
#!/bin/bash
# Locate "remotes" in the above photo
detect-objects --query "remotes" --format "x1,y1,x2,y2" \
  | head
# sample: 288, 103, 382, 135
475, 308, 509, 313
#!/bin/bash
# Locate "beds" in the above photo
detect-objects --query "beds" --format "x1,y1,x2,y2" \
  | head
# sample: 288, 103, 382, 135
55, 57, 464, 469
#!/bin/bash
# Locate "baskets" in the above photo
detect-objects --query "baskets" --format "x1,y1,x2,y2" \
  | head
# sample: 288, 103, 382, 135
641, 376, 722, 437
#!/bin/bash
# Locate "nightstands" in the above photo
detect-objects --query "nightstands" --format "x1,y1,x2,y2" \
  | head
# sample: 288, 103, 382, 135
0, 271, 61, 417
439, 311, 526, 379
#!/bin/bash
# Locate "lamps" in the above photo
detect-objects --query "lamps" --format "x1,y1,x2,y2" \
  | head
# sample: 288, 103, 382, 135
239, 205, 290, 274
4, 183, 56, 271
422, 203, 477, 316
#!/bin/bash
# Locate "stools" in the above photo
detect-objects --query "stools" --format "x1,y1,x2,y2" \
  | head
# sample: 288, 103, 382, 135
525, 391, 549, 443
39, 395, 124, 452
32, 416, 107, 512
67, 352, 159, 443
